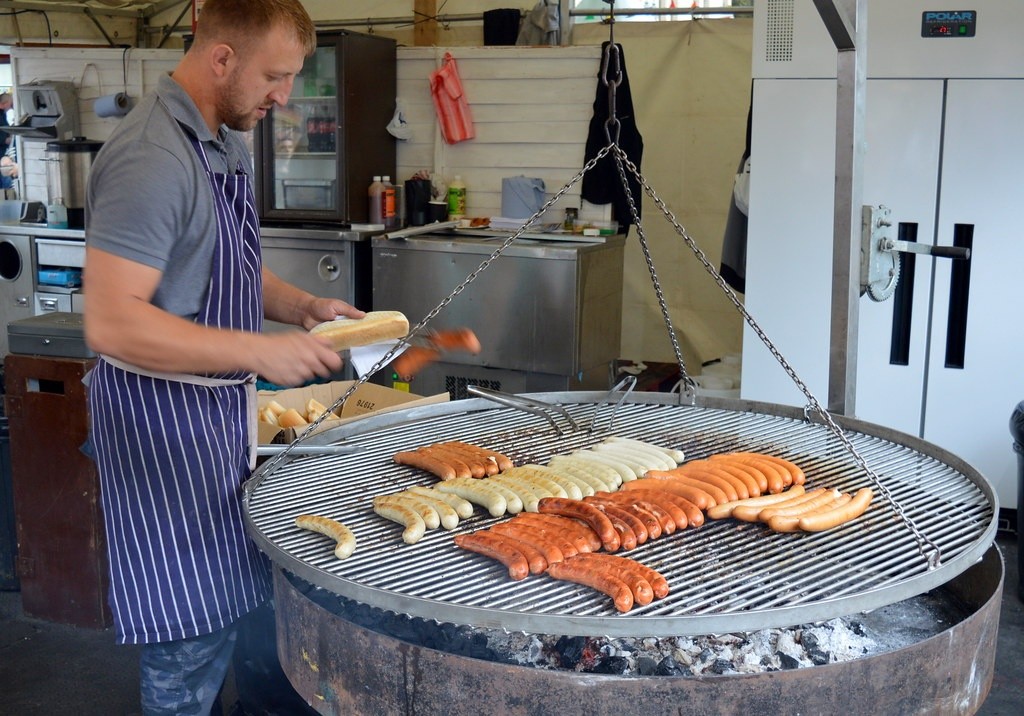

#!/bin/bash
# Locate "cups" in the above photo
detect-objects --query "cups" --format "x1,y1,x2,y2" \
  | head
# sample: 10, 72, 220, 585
426, 199, 449, 237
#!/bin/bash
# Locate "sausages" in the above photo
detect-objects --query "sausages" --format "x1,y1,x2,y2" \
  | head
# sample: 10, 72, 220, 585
294, 327, 873, 612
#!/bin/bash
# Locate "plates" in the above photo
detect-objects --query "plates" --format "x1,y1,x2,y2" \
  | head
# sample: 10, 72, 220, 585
456, 223, 492, 229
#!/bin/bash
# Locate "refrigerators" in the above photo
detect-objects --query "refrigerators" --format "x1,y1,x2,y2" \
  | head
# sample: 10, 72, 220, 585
253, 17, 398, 228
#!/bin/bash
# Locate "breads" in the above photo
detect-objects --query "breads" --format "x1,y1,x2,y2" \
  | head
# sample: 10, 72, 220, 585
257, 310, 408, 428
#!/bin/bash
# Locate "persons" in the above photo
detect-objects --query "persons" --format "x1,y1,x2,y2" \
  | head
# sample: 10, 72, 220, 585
82, 0, 367, 715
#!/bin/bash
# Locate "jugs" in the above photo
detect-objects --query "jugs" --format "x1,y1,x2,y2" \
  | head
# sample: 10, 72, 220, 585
0, 197, 29, 224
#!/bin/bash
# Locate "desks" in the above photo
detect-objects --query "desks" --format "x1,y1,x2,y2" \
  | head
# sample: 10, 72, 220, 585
254, 220, 368, 387
371, 228, 621, 402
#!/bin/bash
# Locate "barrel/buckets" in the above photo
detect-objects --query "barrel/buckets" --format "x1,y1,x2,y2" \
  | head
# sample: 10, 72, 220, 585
40, 132, 108, 229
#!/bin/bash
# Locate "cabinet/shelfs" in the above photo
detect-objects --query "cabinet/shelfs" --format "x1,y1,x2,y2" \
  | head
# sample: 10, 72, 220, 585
251, 28, 399, 227
741, 79, 1021, 513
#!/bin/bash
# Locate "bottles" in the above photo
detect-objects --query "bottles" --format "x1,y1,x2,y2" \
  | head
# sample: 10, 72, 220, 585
367, 176, 387, 225
283, 102, 336, 152
381, 175, 396, 226
299, 48, 318, 97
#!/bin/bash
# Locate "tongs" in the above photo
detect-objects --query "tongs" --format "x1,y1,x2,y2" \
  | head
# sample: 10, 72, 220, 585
255, 440, 366, 455
586, 374, 639, 434
463, 384, 578, 437
